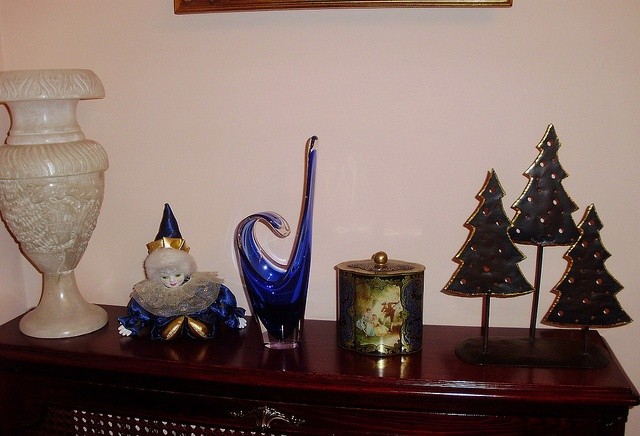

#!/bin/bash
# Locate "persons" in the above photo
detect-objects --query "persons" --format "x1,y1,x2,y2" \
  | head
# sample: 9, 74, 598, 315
117, 204, 246, 345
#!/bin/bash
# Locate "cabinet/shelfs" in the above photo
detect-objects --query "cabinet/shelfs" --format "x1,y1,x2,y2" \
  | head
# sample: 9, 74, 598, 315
0, 298, 640, 436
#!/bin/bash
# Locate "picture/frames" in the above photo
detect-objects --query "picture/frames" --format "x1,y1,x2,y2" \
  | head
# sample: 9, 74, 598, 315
173, 0, 513, 16
